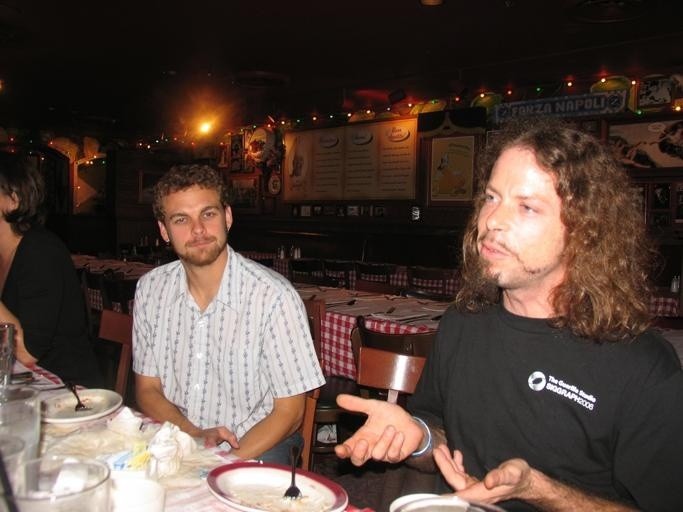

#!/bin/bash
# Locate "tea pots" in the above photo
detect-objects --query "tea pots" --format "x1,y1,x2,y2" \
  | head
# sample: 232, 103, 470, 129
670, 275, 680, 294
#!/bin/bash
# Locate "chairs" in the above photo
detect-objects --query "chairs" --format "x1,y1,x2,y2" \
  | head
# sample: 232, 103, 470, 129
303, 299, 327, 368
297, 359, 325, 473
97, 308, 132, 406
73, 253, 467, 401
345, 315, 440, 408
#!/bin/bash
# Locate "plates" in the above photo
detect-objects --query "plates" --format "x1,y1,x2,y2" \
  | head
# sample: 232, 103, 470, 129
39, 387, 121, 422
388, 494, 505, 512
206, 461, 356, 512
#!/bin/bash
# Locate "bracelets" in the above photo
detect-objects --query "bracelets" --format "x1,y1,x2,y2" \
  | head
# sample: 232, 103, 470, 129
411, 414, 433, 458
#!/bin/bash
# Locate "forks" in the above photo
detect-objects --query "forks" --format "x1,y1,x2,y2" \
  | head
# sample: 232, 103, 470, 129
66, 382, 91, 411
286, 445, 303, 500
366, 306, 396, 317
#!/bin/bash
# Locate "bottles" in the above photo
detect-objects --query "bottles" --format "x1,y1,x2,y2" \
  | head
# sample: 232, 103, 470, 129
277, 244, 300, 259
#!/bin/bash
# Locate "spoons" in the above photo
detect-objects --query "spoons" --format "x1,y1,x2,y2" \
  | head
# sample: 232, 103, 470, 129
325, 299, 355, 309
401, 314, 442, 325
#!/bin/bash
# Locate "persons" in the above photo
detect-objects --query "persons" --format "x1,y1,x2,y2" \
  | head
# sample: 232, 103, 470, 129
332, 113, 680, 512
0, 158, 98, 388
128, 162, 328, 460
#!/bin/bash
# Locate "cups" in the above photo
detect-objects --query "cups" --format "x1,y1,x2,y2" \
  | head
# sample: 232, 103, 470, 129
11, 455, 112, 511
0, 438, 25, 511
0, 322, 15, 401
0, 384, 41, 492
